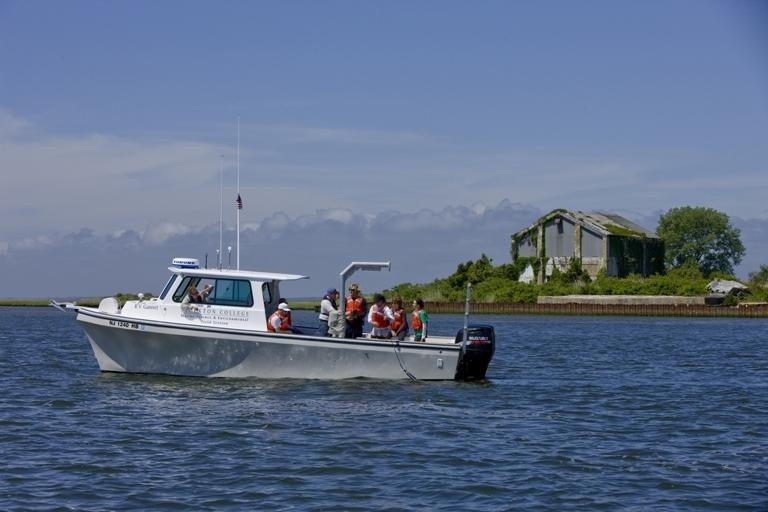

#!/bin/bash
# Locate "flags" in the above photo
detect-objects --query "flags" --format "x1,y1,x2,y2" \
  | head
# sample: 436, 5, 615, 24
236, 194, 242, 209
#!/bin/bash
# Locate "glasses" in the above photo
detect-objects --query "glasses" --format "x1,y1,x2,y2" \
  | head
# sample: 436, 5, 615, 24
348, 288, 356, 292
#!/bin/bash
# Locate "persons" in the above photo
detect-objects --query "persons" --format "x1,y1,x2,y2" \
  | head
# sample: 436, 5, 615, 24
267, 302, 293, 334
247, 283, 270, 308
275, 298, 305, 334
318, 282, 430, 343
182, 284, 214, 312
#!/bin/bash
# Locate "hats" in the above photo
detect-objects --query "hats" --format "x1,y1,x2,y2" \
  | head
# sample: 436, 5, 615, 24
373, 294, 383, 303
326, 287, 338, 296
278, 302, 291, 313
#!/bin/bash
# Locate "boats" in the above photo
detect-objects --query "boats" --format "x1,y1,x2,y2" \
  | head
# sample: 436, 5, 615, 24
48, 258, 496, 381
